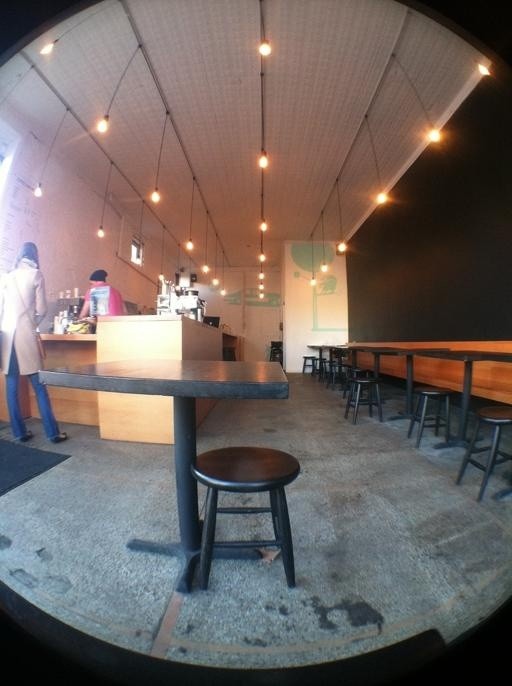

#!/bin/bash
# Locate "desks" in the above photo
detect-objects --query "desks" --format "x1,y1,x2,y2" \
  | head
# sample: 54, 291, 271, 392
307, 344, 512, 455
38, 360, 289, 595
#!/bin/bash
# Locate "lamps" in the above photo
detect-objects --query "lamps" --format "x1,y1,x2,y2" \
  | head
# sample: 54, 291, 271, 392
212, 222, 271, 298
151, 114, 168, 203
97, 44, 141, 132
337, 178, 348, 254
257, 1, 272, 56
310, 235, 318, 286
41, 2, 118, 54
407, 13, 492, 78
201, 213, 210, 274
34, 108, 69, 198
258, 75, 269, 168
97, 163, 112, 239
392, 54, 441, 142
361, 115, 389, 204
319, 211, 329, 274
186, 180, 195, 250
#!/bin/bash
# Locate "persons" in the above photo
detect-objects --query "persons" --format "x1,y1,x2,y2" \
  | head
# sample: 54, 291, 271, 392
1, 241, 69, 445
78, 269, 128, 323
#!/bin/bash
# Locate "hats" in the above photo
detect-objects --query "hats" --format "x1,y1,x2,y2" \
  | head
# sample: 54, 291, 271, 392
89, 270, 107, 280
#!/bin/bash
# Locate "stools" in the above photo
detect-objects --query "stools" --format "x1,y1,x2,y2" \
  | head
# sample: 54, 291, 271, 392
303, 355, 512, 500
190, 446, 301, 590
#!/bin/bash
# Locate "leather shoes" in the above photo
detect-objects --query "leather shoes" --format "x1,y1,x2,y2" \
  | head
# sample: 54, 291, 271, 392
51, 432, 67, 443
21, 431, 32, 442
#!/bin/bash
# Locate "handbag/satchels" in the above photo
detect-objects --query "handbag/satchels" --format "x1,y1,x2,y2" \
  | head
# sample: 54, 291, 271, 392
37, 332, 47, 360
